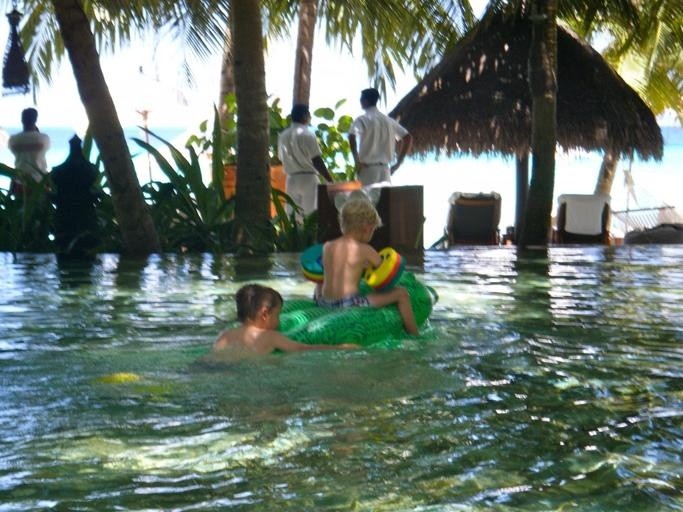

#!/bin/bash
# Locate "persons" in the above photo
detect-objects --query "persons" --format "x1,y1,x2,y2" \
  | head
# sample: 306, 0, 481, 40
347, 88, 413, 187
313, 199, 420, 336
186, 283, 361, 374
6, 107, 50, 194
276, 103, 334, 221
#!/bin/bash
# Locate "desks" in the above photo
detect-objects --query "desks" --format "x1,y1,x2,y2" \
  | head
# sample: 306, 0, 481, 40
318, 185, 424, 273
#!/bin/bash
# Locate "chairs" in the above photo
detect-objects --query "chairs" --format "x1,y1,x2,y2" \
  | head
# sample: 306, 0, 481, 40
553, 192, 612, 246
447, 191, 502, 245
